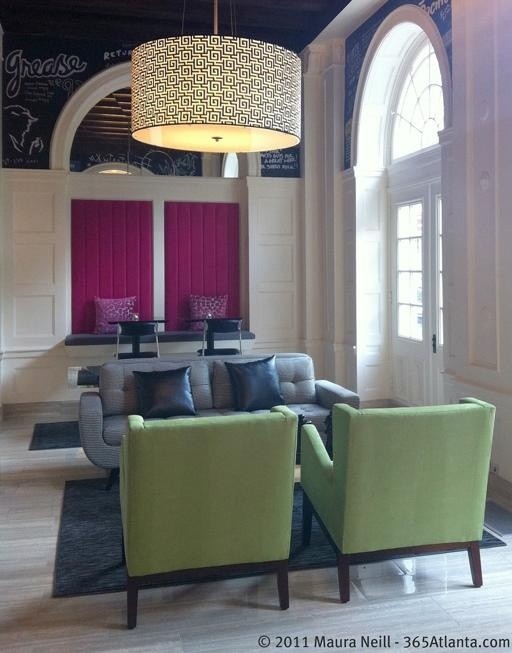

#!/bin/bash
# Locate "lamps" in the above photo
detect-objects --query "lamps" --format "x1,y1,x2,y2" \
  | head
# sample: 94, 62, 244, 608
130, 0, 301, 153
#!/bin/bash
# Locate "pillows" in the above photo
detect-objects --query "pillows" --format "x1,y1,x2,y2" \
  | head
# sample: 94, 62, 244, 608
93, 295, 136, 334
132, 366, 197, 418
224, 354, 284, 411
188, 294, 230, 332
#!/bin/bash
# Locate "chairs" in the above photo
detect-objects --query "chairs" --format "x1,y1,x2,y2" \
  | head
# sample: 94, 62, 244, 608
118, 406, 300, 627
299, 397, 496, 603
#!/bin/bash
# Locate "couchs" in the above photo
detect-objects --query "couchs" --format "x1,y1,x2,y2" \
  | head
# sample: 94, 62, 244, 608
78, 352, 359, 470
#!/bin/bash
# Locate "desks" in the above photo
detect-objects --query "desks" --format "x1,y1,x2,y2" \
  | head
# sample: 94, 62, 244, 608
185, 315, 245, 356
105, 318, 167, 359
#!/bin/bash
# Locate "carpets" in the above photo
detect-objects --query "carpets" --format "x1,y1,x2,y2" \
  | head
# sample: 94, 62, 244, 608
50, 478, 506, 598
28, 421, 82, 451
